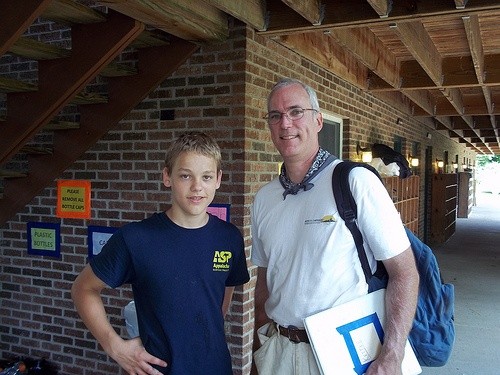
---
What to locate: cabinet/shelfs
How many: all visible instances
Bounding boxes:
[381,175,420,237]
[431,172,458,244]
[457,172,474,219]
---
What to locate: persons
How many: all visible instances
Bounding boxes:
[71,131,250,375]
[251,78,420,375]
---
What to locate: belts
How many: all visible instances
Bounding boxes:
[274,322,310,344]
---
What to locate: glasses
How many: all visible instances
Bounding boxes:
[263,106,319,125]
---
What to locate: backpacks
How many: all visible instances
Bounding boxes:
[332,161,455,367]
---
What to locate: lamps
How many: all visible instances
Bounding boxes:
[452,161,458,169]
[408,153,419,167]
[357,142,372,163]
[436,158,444,167]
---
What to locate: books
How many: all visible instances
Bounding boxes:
[303,289,422,375]
